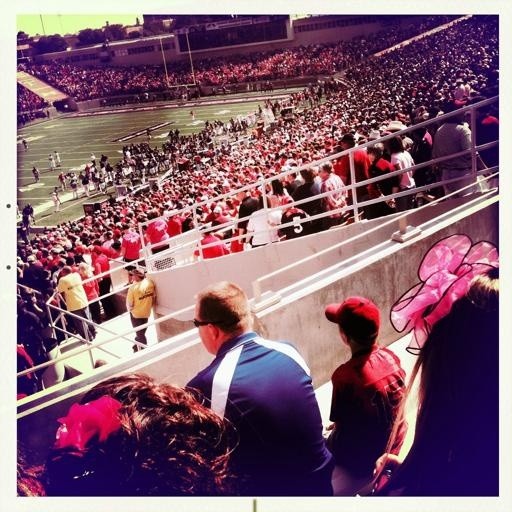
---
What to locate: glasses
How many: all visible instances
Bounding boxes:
[192,318,210,327]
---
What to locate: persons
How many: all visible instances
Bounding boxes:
[19,58,69,82]
[69,58,170,82]
[356,270,500,497]
[93,188,143,262]
[79,263,101,325]
[144,155,254,259]
[115,155,143,188]
[48,154,55,171]
[17,82,51,125]
[53,82,170,106]
[57,266,96,341]
[185,280,335,497]
[32,167,39,183]
[57,211,93,264]
[170,50,254,101]
[22,203,35,225]
[126,267,157,352]
[162,117,254,155]
[324,295,408,476]
[22,138,29,152]
[79,152,115,188]
[91,246,116,318]
[17,372,240,497]
[66,174,80,200]
[54,151,61,167]
[58,171,67,191]
[50,186,62,211]
[121,127,162,155]
[190,110,196,120]
[17,225,57,371]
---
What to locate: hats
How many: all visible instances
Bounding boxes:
[384,120,408,131]
[325,295,382,341]
[129,268,145,277]
